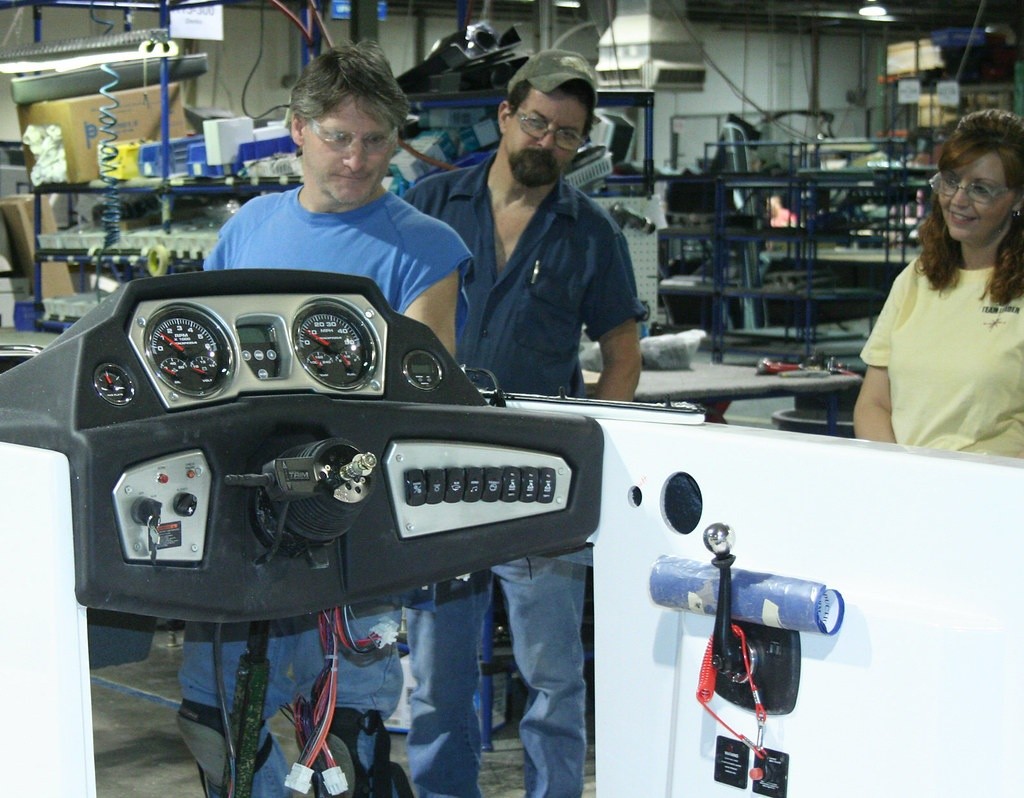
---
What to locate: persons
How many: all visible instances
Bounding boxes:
[854,105,1024,457]
[173,42,475,796]
[763,194,797,252]
[398,47,647,798]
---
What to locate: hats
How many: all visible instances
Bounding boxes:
[507,49,598,110]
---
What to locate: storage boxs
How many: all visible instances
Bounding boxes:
[1,194,76,298]
[16,84,189,188]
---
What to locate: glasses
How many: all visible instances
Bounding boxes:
[929,172,1023,203]
[509,105,585,151]
[304,115,397,154]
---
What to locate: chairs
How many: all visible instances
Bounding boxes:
[771,409,855,437]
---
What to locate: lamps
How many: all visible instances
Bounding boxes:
[858,1,888,19]
[0,28,181,74]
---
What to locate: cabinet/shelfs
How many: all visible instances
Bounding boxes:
[882,48,1024,251]
[714,171,907,363]
[28,177,304,335]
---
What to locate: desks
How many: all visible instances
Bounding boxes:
[577,364,863,438]
[1,328,64,355]
